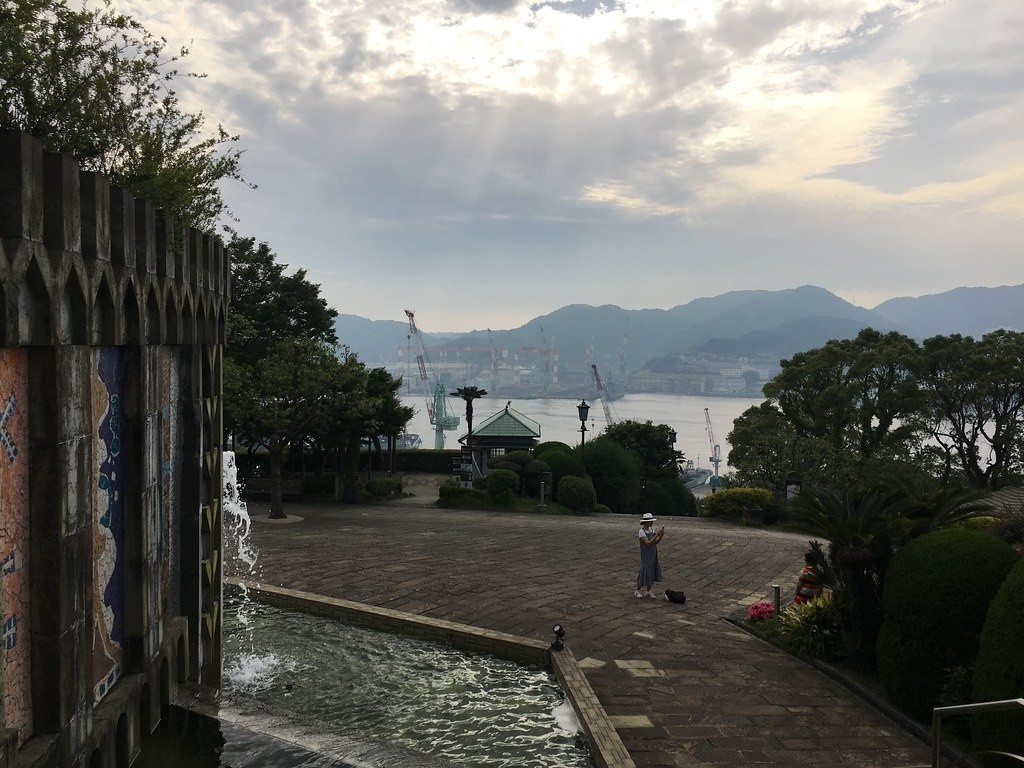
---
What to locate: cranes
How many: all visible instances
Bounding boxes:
[404,307,460,449]
[487,325,566,397]
[704,407,724,487]
[592,365,620,428]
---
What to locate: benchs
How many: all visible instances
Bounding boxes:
[242,477,305,504]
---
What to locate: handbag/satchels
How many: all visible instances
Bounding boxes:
[665,589,686,604]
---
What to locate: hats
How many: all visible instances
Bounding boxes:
[639,513,657,523]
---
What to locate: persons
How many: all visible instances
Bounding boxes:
[793,552,824,606]
[635,513,665,599]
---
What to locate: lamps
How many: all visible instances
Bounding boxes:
[551,624,565,651]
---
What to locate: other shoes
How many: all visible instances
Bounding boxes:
[635,591,643,597]
[645,591,655,598]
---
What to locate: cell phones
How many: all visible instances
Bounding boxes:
[662,525,665,530]
